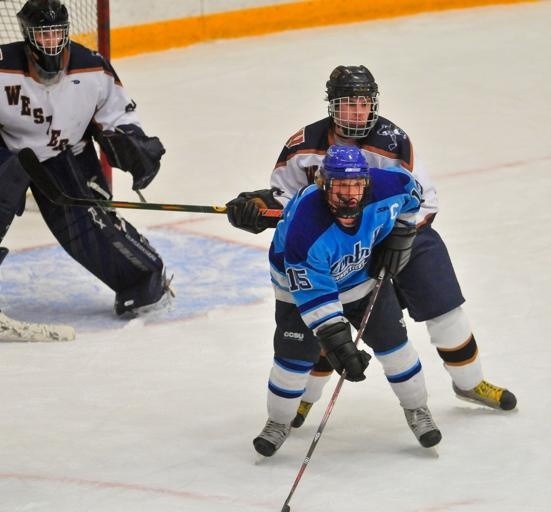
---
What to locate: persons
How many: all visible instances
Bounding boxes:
[251,143,443,457]
[0,0,177,322]
[226,62,519,430]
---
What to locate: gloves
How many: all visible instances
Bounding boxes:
[316,320,371,382]
[368,226,416,282]
[225,187,283,235]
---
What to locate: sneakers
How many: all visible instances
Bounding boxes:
[253,417,294,455]
[401,404,442,448]
[291,399,317,428]
[451,377,520,410]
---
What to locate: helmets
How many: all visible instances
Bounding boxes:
[325,63,380,138]
[317,142,375,221]
[17,0,69,64]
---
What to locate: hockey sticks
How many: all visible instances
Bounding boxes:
[282,265,383,512]
[18,147,282,219]
